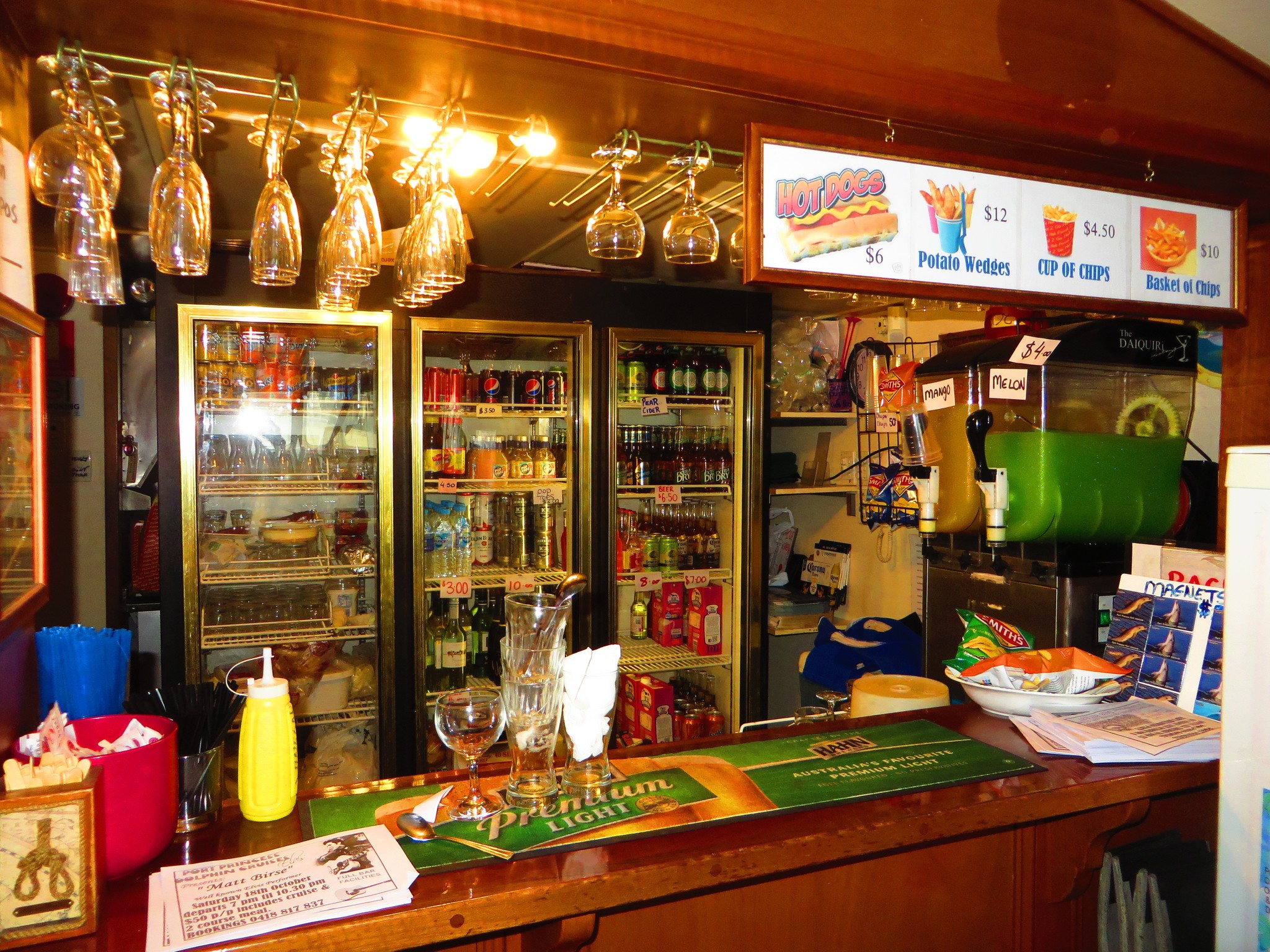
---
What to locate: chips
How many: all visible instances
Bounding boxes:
[878,360,923,431]
[943,608,1036,677]
[959,646,1135,694]
[865,463,919,533]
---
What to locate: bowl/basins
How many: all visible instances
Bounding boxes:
[11,713,180,886]
[1148,224,1188,264]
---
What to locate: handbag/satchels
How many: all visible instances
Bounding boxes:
[803,612,925,694]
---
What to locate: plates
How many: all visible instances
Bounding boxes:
[840,702,852,714]
[945,666,1121,717]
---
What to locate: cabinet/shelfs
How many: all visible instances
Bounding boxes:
[768,410,858,640]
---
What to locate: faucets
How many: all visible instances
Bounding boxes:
[903,409,943,542]
[964,407,1011,561]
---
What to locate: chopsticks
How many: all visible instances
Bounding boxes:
[121,679,247,819]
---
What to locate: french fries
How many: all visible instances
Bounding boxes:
[920,178,976,220]
[1042,204,1078,223]
[1145,218,1189,260]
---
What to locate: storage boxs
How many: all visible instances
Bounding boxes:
[1,765,104,950]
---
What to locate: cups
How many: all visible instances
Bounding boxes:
[178,741,224,833]
[881,354,943,466]
[202,431,375,636]
[793,707,835,724]
[847,679,857,709]
[497,593,621,805]
[1044,216,1074,257]
[927,198,974,254]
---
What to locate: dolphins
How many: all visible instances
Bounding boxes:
[1107,596,1222,721]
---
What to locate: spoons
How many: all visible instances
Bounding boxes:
[397,813,514,862]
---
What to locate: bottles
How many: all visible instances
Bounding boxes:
[424,414,734,758]
[228,647,299,822]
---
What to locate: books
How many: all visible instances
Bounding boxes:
[1005,689,1221,766]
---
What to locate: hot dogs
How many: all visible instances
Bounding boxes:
[780,194,898,262]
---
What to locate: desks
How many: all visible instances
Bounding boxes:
[0,707,1219,952]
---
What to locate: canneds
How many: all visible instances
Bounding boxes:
[456,492,557,570]
[616,360,648,404]
[423,367,568,413]
[674,698,725,741]
[196,321,372,413]
[631,532,678,577]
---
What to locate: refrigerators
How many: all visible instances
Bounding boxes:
[151,288,766,795]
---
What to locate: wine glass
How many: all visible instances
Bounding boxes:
[434,687,504,824]
[816,690,849,714]
[26,54,746,314]
